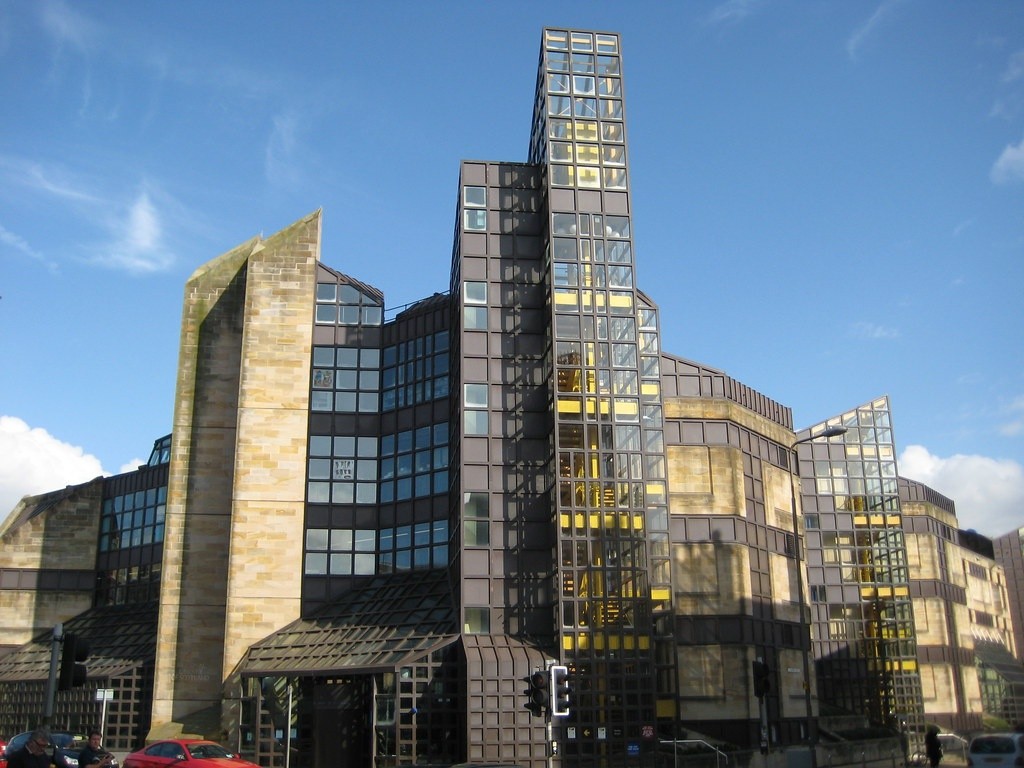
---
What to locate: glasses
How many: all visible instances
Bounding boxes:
[33,739,47,749]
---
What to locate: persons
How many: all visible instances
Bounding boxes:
[6,728,50,768]
[78,731,113,768]
[925,727,943,768]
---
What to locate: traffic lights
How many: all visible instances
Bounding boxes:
[550,665,572,716]
[531,671,549,708]
[523,675,542,717]
[52,631,91,694]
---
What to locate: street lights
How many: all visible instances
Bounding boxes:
[788,425,850,768]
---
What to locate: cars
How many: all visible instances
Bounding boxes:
[4,729,120,768]
[123,739,264,768]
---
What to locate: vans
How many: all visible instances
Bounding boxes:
[966,734,1023,768]
